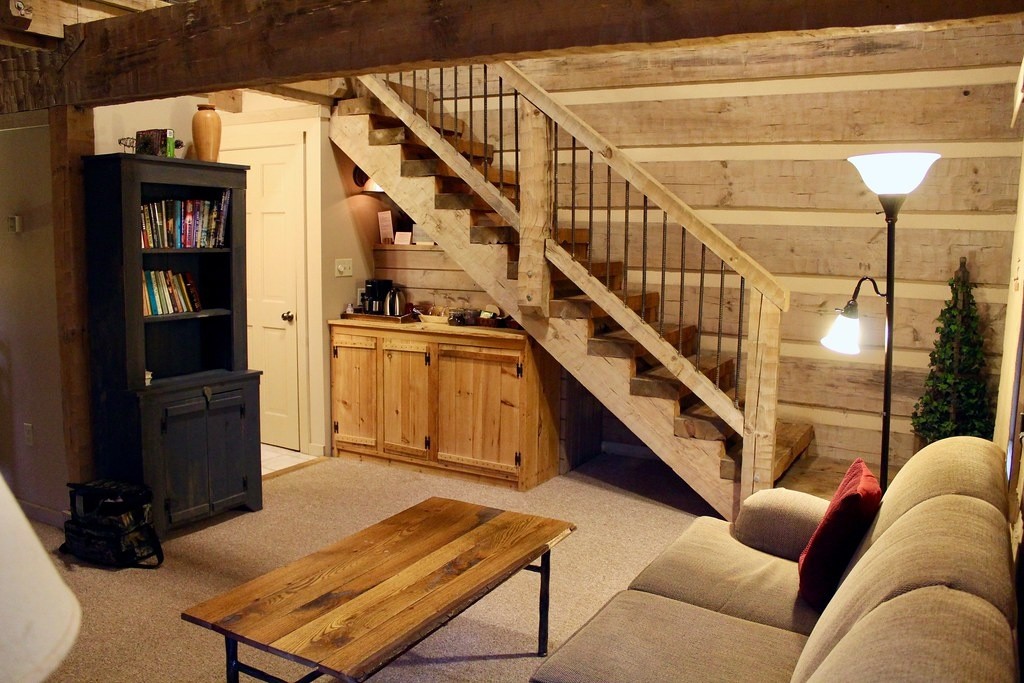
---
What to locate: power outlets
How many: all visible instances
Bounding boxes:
[357,288,365,306]
[24,423,32,446]
[335,259,352,277]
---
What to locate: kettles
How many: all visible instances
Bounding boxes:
[384,287,407,316]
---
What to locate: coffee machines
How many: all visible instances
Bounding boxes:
[360,279,392,314]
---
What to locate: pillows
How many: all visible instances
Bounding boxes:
[800,458,883,614]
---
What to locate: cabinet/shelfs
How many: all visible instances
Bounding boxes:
[81,152,265,543]
[328,317,560,492]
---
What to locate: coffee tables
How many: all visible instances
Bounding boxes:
[181,496,577,683]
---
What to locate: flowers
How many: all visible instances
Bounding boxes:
[175,140,184,149]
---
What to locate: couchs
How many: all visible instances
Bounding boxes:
[529,436,1020,683]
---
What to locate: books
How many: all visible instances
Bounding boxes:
[140,190,230,316]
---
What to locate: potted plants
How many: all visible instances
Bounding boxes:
[910,257,999,456]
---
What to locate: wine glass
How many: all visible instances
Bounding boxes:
[427,291,440,316]
[440,293,452,317]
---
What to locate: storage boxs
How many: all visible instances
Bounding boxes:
[136,130,174,158]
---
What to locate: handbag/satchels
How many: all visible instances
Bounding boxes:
[58,475,165,570]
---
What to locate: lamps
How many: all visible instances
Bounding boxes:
[819,153,944,495]
[353,165,384,192]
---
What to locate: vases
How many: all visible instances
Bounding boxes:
[192,104,222,162]
[174,148,183,157]
